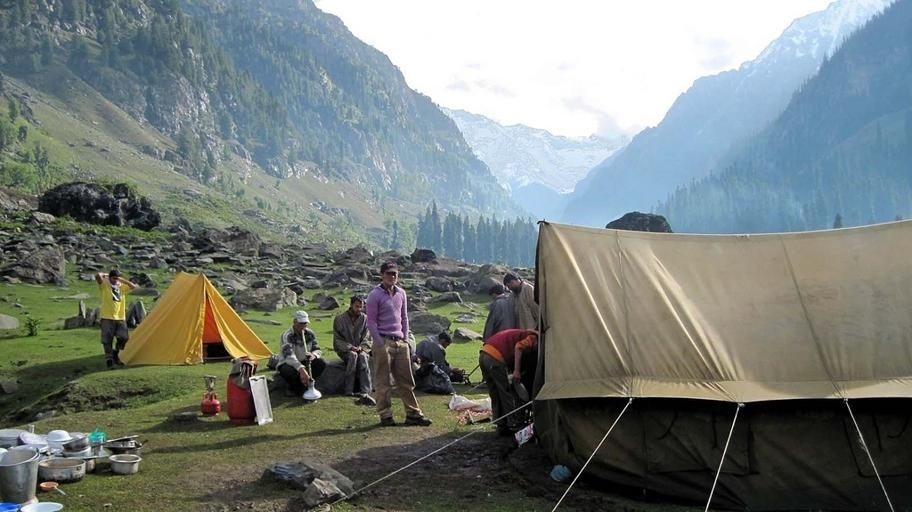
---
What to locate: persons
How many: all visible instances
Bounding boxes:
[333,294,374,398]
[94,269,137,367]
[503,271,540,329]
[416,333,464,382]
[276,310,326,403]
[479,284,516,381]
[479,328,539,437]
[365,261,432,425]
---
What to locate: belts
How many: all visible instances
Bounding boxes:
[379,332,403,342]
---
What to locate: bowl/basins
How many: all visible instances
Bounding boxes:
[173,412,197,421]
[0,429,146,482]
[0,499,65,512]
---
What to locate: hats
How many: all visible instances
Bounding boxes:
[380,262,399,274]
[293,310,311,325]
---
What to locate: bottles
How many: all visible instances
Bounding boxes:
[302,361,323,402]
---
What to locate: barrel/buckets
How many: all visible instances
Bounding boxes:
[88,431,105,449]
[0,448,41,505]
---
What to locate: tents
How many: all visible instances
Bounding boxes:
[117,271,273,368]
[534,218,911,512]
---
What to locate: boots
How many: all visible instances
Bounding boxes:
[105,350,124,371]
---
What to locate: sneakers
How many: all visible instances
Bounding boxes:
[381,416,395,426]
[360,394,376,405]
[404,415,432,427]
